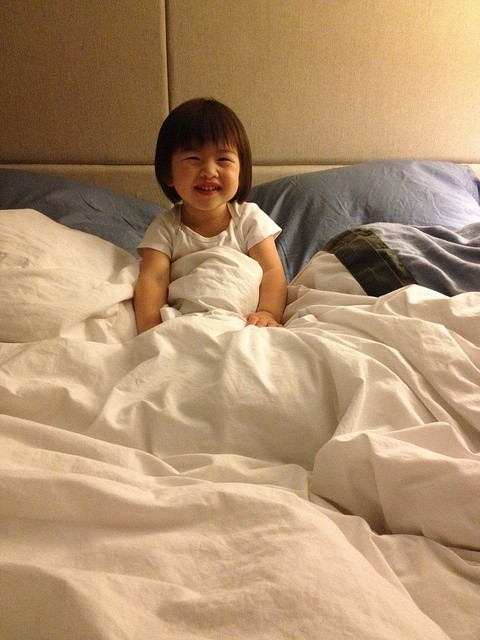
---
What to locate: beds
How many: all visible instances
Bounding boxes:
[0,164,480,640]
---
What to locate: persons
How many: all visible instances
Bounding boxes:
[134,98,288,334]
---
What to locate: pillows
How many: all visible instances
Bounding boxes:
[0,166,167,260]
[243,161,480,285]
